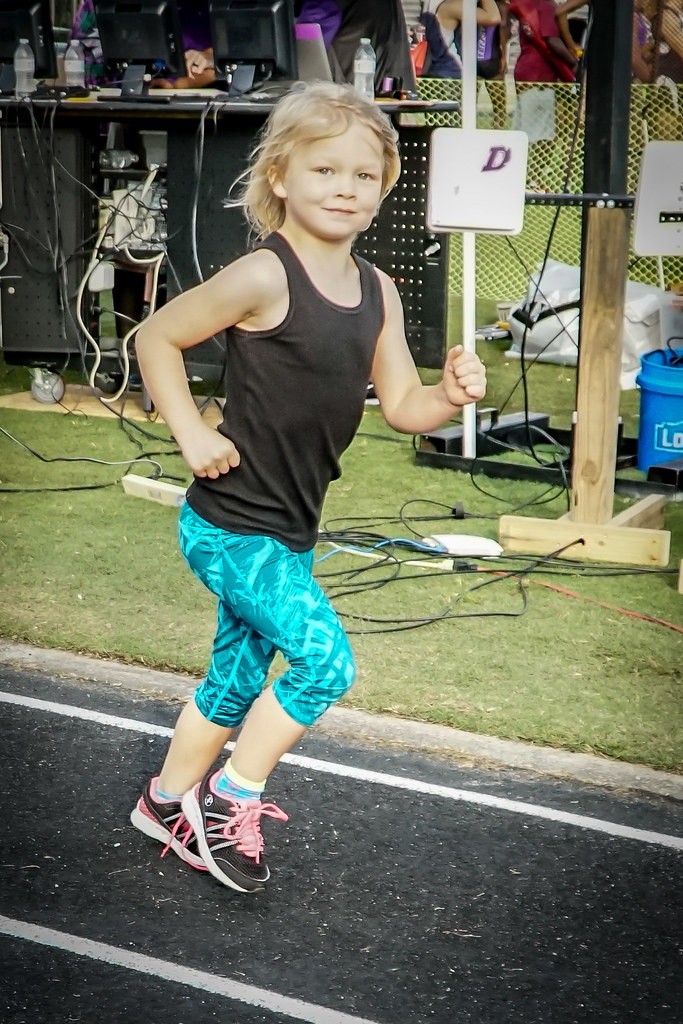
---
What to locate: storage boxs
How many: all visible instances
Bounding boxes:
[657,290,683,350]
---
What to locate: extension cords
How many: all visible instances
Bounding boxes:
[121,474,195,507]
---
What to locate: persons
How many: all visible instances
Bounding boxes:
[130,76,486,892]
[147,1,682,198]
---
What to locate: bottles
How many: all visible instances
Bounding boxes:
[352,37,376,101]
[98,149,139,169]
[65,40,85,89]
[14,39,36,100]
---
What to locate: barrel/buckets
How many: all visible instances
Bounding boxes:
[634,347,683,475]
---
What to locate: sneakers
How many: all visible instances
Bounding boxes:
[181,769,289,893]
[131,777,209,871]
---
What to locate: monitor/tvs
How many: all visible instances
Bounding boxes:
[0,0,59,80]
[208,0,300,81]
[93,0,189,78]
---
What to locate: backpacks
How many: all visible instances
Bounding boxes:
[410,0,449,77]
[477,22,501,79]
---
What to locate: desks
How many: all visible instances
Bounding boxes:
[0,98,463,414]
